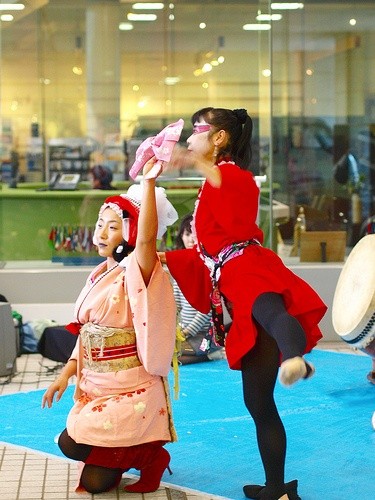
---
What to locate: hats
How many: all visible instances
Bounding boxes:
[91,184,179,249]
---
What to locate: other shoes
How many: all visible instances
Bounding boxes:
[242,479,301,500]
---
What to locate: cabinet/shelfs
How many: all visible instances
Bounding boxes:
[49,138,90,180]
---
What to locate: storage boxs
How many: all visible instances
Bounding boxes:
[300,230,347,262]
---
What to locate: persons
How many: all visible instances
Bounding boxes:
[41,155,179,494]
[86,164,117,190]
[155,107,328,500]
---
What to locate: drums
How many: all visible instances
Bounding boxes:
[331,233,375,357]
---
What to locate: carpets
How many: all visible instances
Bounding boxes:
[0,349,375,500]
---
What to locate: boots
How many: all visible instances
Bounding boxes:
[84,441,173,493]
